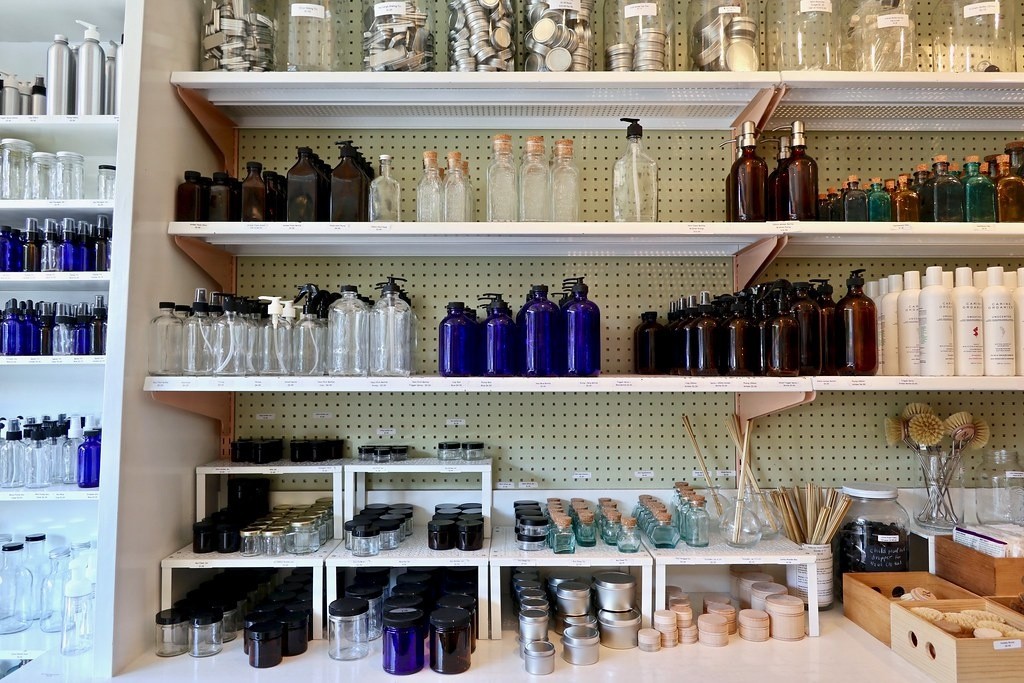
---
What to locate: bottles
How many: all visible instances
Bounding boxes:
[838,480,909,601]
[326,286,377,377]
[515,286,559,376]
[553,139,580,222]
[0,529,97,654]
[486,134,516,222]
[544,480,781,554]
[44,19,124,116]
[146,302,221,375]
[521,133,552,222]
[176,147,330,222]
[629,292,721,375]
[819,141,1024,223]
[417,149,475,223]
[368,155,401,222]
[435,300,478,377]
[976,447,1024,527]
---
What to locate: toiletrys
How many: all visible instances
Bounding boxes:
[631,264,1024,377]
[724,119,1024,224]
[173,140,376,223]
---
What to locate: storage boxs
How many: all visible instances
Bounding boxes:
[842,572,982,648]
[891,600,1024,683]
[934,534,1024,596]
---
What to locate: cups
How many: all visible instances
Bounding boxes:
[1,138,34,200]
[152,569,313,667]
[56,153,84,200]
[853,0,915,70]
[767,0,842,72]
[98,164,115,200]
[201,0,762,72]
[329,566,478,674]
[192,478,332,556]
[347,502,485,555]
[29,152,55,199]
[786,543,837,610]
[513,499,549,550]
[931,0,1014,73]
[229,439,485,463]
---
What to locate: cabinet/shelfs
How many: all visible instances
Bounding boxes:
[0,71,1024,683]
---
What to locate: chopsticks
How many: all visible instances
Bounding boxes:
[772,484,852,544]
